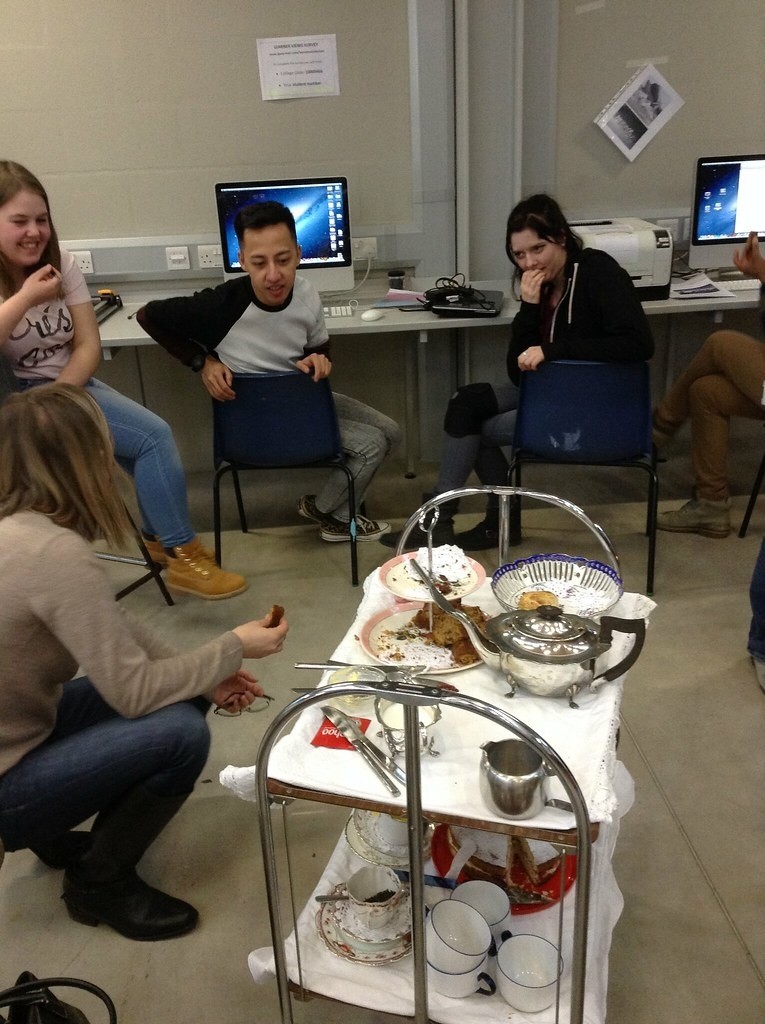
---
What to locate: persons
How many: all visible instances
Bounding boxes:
[0,161,248,599]
[137,201,402,544]
[654,231,765,539]
[0,383,287,940]
[378,192,655,549]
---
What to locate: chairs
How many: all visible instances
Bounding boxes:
[0,350,176,610]
[504,357,658,596]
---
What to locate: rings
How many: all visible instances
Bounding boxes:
[522,352,526,356]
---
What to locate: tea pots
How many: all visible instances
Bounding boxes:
[448,606,646,709]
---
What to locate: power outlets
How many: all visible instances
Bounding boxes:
[684,218,690,240]
[198,244,222,269]
[73,251,94,274]
[352,237,378,261]
[165,246,190,270]
[657,219,679,241]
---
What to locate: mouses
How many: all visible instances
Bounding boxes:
[361,310,384,322]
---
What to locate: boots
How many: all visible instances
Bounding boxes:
[378,494,456,549]
[28,831,89,870]
[62,781,199,942]
[455,493,522,550]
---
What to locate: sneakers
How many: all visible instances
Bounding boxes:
[652,427,678,462]
[140,527,248,600]
[657,486,733,538]
[298,495,391,542]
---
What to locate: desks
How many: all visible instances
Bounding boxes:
[81,268,761,481]
[209,371,365,587]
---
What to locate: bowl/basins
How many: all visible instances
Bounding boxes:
[491,552,624,617]
[326,664,386,714]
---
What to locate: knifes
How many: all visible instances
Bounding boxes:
[411,558,471,624]
[289,660,460,796]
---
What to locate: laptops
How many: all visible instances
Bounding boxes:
[432,290,504,316]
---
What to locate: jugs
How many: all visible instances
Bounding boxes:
[480,738,573,819]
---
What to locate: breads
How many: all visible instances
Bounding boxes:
[405,543,472,584]
[412,597,489,665]
[269,604,285,627]
[518,591,559,611]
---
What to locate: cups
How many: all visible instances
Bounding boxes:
[427,953,496,999]
[347,864,402,930]
[496,931,564,1013]
[424,899,492,974]
[448,880,510,940]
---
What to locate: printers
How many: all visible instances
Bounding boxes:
[571,218,673,300]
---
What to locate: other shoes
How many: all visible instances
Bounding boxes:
[752,654,765,694]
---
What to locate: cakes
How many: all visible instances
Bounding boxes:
[448,825,564,885]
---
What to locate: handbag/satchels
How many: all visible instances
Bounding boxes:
[0,971,117,1024]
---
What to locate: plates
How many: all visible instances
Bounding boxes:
[431,824,577,915]
[315,882,426,968]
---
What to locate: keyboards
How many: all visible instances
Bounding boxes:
[323,306,352,317]
[715,279,763,292]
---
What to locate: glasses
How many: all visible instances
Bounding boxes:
[213,692,275,717]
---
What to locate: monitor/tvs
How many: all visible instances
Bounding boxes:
[688,154,765,279]
[215,176,355,292]
